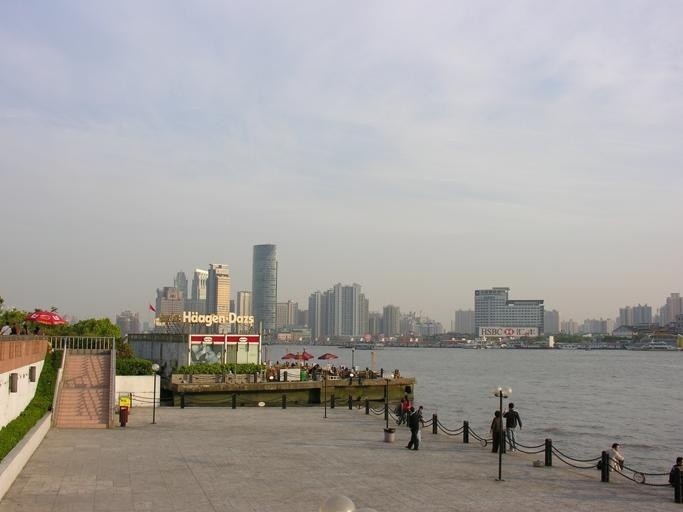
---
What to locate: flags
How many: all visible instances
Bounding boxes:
[148,304,156,312]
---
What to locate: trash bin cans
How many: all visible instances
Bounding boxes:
[313,369,321,381]
[384,428,396,442]
[300,371,306,381]
[119,406,128,426]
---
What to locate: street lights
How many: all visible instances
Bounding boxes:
[490,386,512,481]
[151,363,160,424]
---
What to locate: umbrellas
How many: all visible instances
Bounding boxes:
[26,311,64,335]
[281,351,338,370]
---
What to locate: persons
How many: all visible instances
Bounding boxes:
[489,402,523,452]
[669,457,683,487]
[396,395,424,450]
[266,360,374,382]
[601,443,624,471]
[191,344,221,365]
[0,321,41,335]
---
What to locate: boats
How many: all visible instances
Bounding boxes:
[641,341,674,351]
[343,341,383,349]
